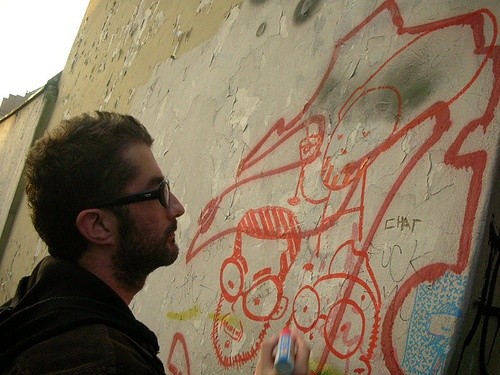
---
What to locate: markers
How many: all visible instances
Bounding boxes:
[274,325,296,374]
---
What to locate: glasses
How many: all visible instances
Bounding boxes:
[81,180,171,214]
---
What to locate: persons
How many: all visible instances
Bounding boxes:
[1,110,311,375]
